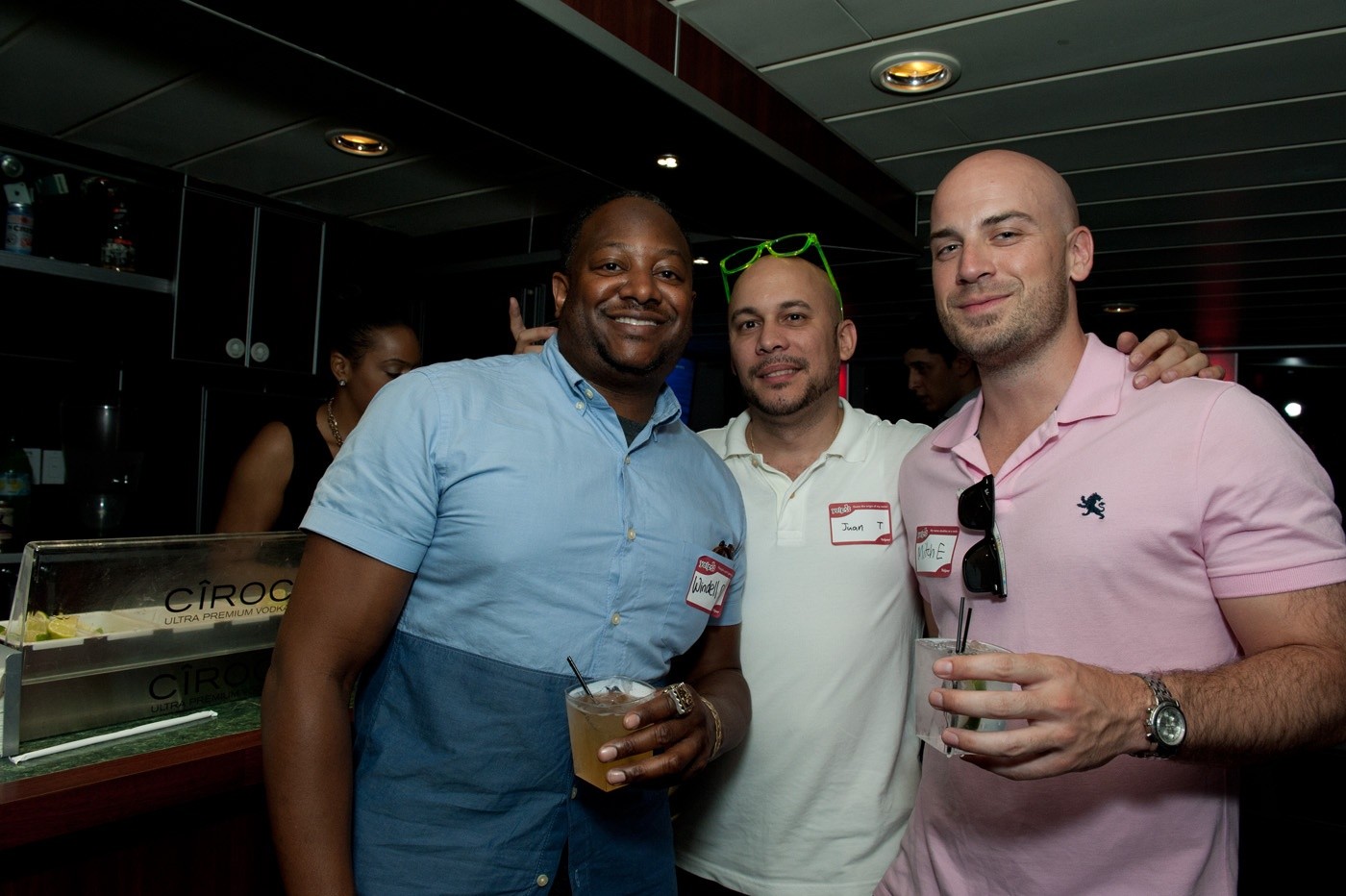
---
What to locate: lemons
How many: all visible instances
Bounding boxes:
[257,589,289,607]
[958,679,987,730]
[19,611,104,642]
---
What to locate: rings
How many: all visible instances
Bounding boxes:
[662,682,695,714]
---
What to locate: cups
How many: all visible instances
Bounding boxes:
[564,675,657,792]
[912,637,1015,758]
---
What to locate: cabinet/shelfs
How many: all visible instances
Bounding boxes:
[170,188,422,378]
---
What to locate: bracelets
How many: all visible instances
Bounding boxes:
[701,697,722,763]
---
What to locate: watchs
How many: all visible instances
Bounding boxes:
[1129,673,1187,759]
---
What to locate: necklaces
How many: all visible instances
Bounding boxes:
[326,397,343,447]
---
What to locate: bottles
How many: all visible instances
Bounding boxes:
[5,202,34,257]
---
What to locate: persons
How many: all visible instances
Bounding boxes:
[261,200,751,896]
[884,310,982,425]
[508,232,1225,896]
[207,316,423,596]
[898,151,1346,896]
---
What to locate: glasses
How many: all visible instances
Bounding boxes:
[719,231,845,317]
[957,473,1008,598]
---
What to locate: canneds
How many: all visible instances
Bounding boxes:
[5,202,34,255]
